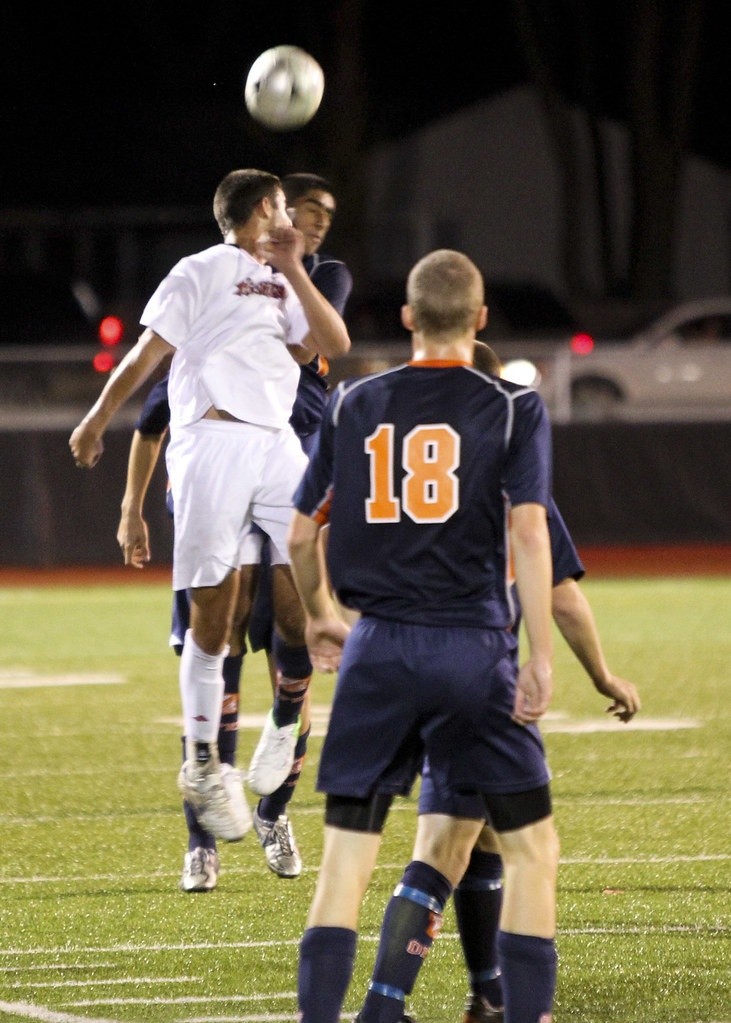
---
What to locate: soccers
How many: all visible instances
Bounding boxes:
[244,46,326,129]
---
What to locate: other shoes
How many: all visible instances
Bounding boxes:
[465,995,504,1023]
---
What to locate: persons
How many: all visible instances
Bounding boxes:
[69,170,354,890]
[288,248,643,1023]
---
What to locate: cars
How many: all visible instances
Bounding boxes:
[538,300,731,427]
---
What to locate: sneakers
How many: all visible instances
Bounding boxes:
[182,846,217,891]
[177,759,251,842]
[248,708,302,796]
[252,804,302,878]
